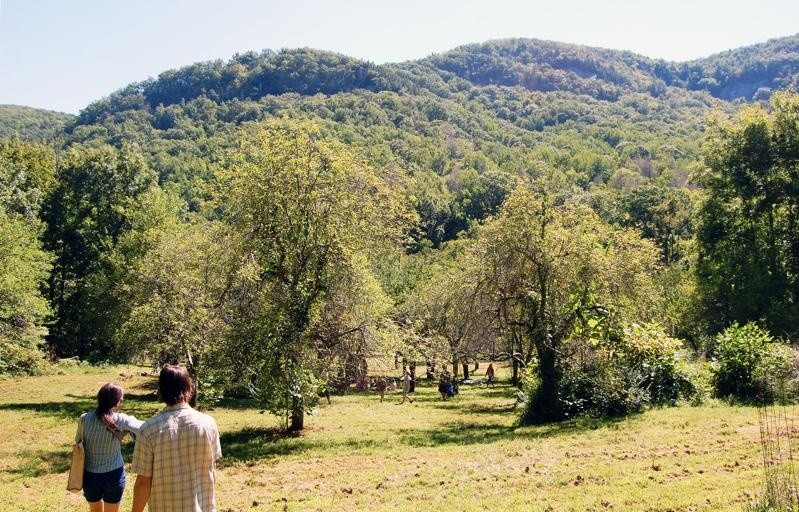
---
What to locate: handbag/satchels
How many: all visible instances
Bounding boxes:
[66,440,86,494]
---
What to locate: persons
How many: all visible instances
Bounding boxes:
[75,382,145,512]
[324,351,419,405]
[426,351,470,401]
[484,363,495,388]
[132,363,222,512]
